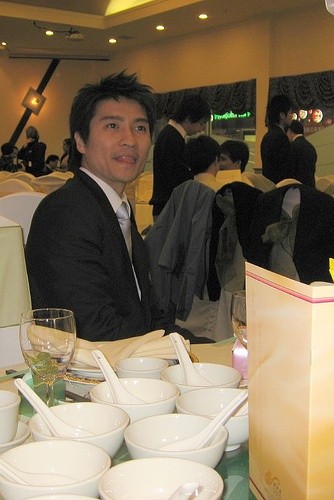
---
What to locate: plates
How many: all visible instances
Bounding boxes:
[0,421,31,453]
[62,341,116,380]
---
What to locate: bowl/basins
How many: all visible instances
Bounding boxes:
[0,440,112,500]
[175,387,248,452]
[123,413,228,469]
[29,402,130,460]
[90,378,180,425]
[160,362,242,395]
[97,457,224,500]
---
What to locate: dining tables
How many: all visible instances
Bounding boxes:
[0,342,249,500]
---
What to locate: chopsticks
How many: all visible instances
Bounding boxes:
[6,369,91,401]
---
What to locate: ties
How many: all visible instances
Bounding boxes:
[116,202,133,267]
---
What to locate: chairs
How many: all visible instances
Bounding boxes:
[0,170,334,370]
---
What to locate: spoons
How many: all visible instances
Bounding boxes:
[14,378,96,437]
[158,388,248,452]
[0,457,79,486]
[90,350,148,405]
[169,333,213,386]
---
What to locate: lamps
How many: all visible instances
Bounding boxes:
[64,30,84,41]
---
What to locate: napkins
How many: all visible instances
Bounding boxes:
[26,324,191,372]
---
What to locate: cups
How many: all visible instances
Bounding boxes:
[231,290,247,349]
[0,390,21,444]
[19,308,76,407]
[115,357,169,380]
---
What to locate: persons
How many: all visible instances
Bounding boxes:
[17,126,46,177]
[11,147,18,165]
[41,155,60,176]
[285,119,318,187]
[0,143,17,173]
[149,96,210,222]
[218,139,254,187]
[60,138,72,172]
[25,68,214,343]
[186,135,224,192]
[260,94,296,183]
